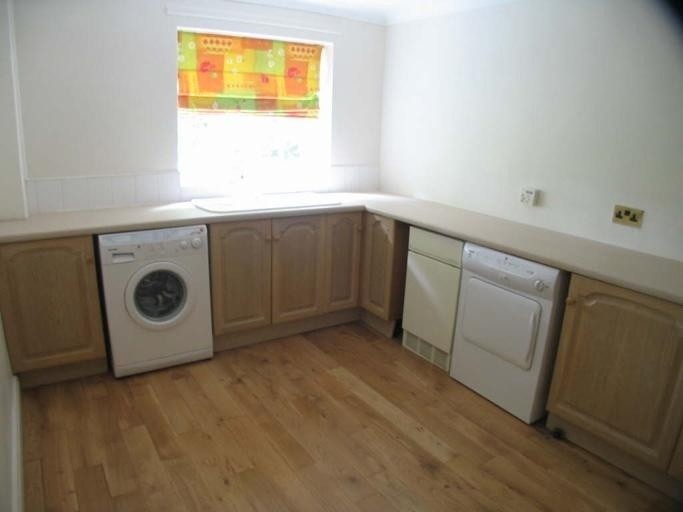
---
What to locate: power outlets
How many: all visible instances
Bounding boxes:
[612,205,644,228]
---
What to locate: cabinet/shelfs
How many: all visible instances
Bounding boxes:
[546,274,683,472]
[325,214,393,317]
[0,236,107,375]
[210,214,326,337]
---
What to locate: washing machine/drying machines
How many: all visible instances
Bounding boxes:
[98,224,214,380]
